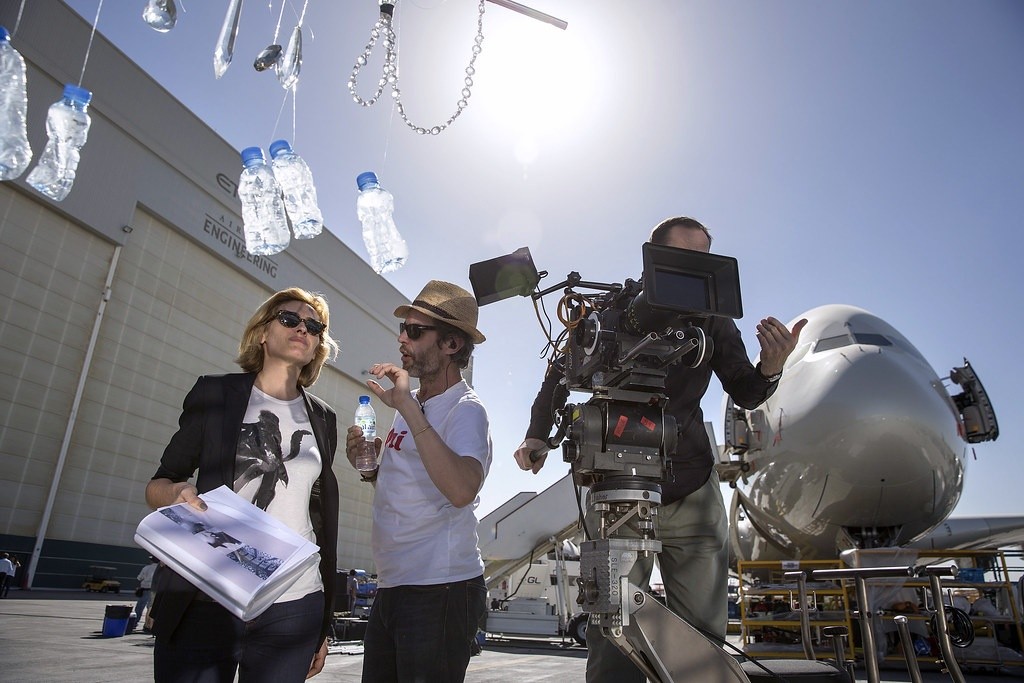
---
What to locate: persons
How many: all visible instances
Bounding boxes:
[346,570,359,623]
[0,553,22,599]
[132,555,161,630]
[359,279,493,683]
[513,215,809,683]
[144,288,340,683]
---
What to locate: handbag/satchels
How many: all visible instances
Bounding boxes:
[135,588,144,598]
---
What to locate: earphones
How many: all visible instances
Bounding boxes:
[450,340,457,349]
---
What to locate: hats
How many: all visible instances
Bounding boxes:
[393,280,486,344]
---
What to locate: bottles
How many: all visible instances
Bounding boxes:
[26,82,92,202]
[238,146,291,256]
[356,171,410,275]
[354,396,378,471]
[0,26,33,181]
[269,139,323,240]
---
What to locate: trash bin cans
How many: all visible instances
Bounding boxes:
[103,603,134,637]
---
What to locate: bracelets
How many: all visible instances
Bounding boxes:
[361,464,378,482]
[414,424,432,439]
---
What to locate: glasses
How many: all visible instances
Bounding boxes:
[400,322,461,341]
[265,309,326,338]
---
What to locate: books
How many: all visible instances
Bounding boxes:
[133,485,320,623]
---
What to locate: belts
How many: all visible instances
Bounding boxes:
[144,588,151,591]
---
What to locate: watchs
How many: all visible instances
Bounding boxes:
[757,361,783,383]
